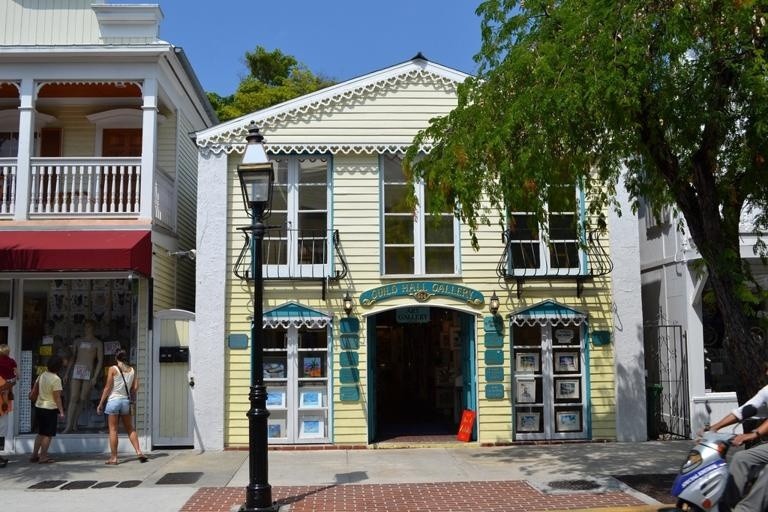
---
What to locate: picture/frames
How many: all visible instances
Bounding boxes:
[433,326,463,410]
[262,356,325,439]
[514,352,580,433]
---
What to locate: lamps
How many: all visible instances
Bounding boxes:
[488,290,499,315]
[343,290,352,317]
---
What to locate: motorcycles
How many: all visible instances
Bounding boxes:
[656,400,767,512]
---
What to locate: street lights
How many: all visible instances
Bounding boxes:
[236,119,280,511]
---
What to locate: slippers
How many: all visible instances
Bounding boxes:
[30,457,55,463]
[106,460,117,464]
[138,454,148,462]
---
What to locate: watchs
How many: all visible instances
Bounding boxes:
[751,429,760,437]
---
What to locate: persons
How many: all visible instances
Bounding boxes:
[60,320,103,433]
[36,319,66,377]
[29,356,65,464]
[102,320,130,368]
[97,349,149,465]
[697,356,768,512]
[0,377,15,468]
[0,344,18,411]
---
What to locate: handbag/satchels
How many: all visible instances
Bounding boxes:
[30,381,39,402]
[131,402,135,415]
